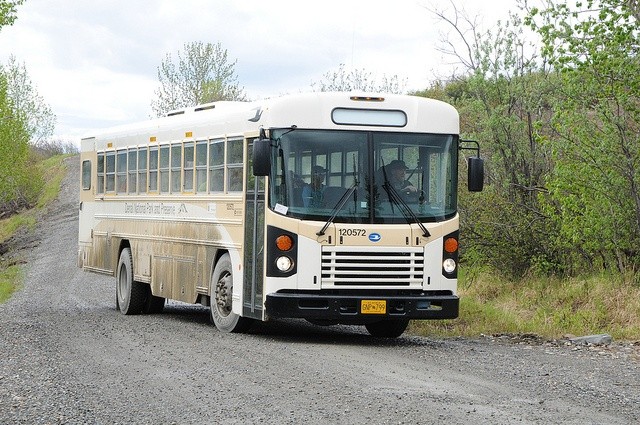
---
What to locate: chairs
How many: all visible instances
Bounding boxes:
[322,186,347,209]
[297,183,308,206]
[281,170,295,199]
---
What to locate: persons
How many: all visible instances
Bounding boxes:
[377,160,418,203]
[301,166,328,207]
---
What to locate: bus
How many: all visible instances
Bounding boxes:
[77,91,484,338]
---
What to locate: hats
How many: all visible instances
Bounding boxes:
[312,166,328,172]
[391,160,409,169]
